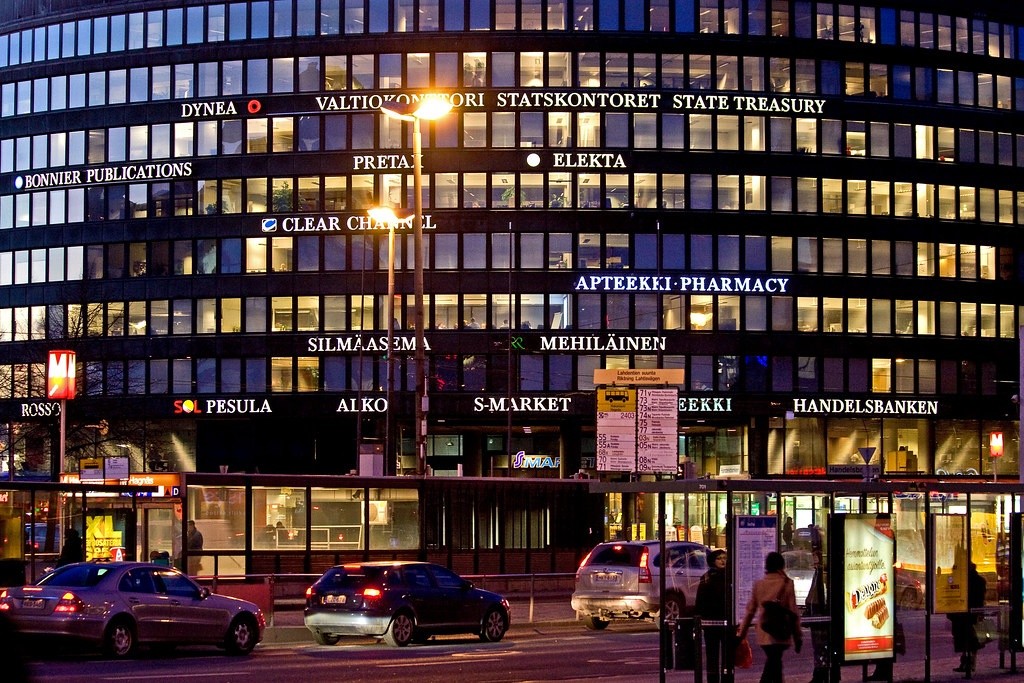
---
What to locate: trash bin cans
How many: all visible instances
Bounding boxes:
[660,616,697,671]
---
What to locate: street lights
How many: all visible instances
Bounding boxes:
[989,430,1004,482]
[410,89,452,475]
[366,206,400,475]
[46,349,77,483]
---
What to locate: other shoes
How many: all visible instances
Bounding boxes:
[867,670,891,680]
[952,654,974,673]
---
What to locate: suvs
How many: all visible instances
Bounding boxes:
[571,538,718,630]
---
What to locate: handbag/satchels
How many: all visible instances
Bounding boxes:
[761,579,800,642]
[894,618,908,655]
[802,604,811,627]
[730,627,753,669]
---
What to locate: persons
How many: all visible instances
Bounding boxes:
[437,317,512,330]
[695,516,985,683]
[181,520,204,578]
[55,527,84,570]
[260,521,290,541]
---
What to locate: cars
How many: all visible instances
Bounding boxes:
[303,560,510,648]
[0,561,265,657]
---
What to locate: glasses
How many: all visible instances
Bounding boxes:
[716,557,727,559]
